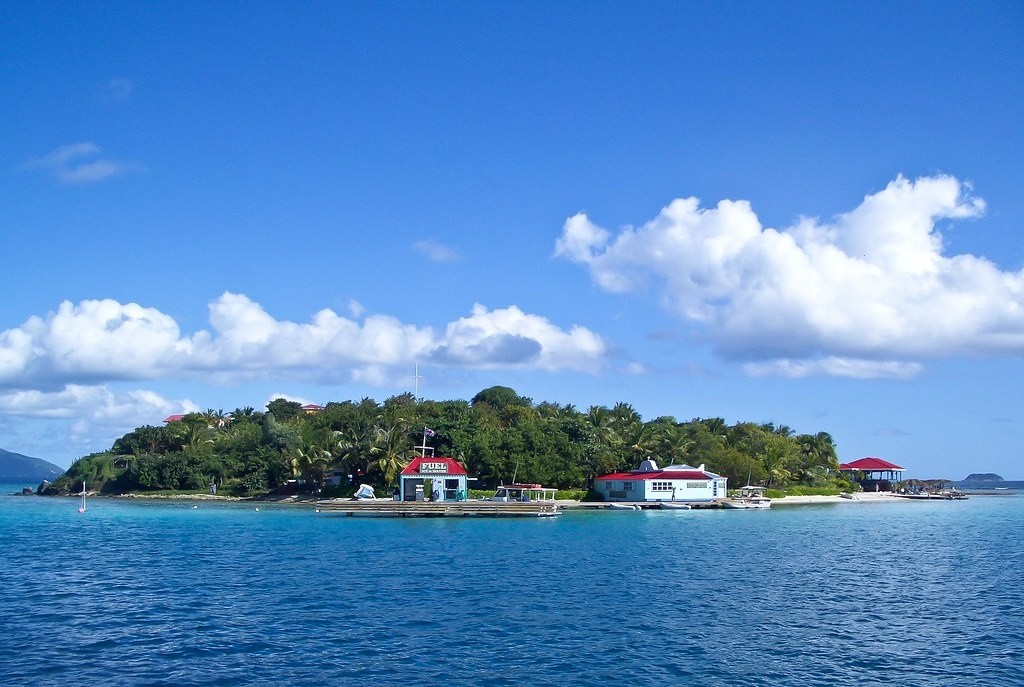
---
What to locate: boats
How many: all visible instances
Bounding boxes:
[477,461,561,515]
[721,469,771,508]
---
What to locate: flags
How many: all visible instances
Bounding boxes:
[424,428,436,437]
